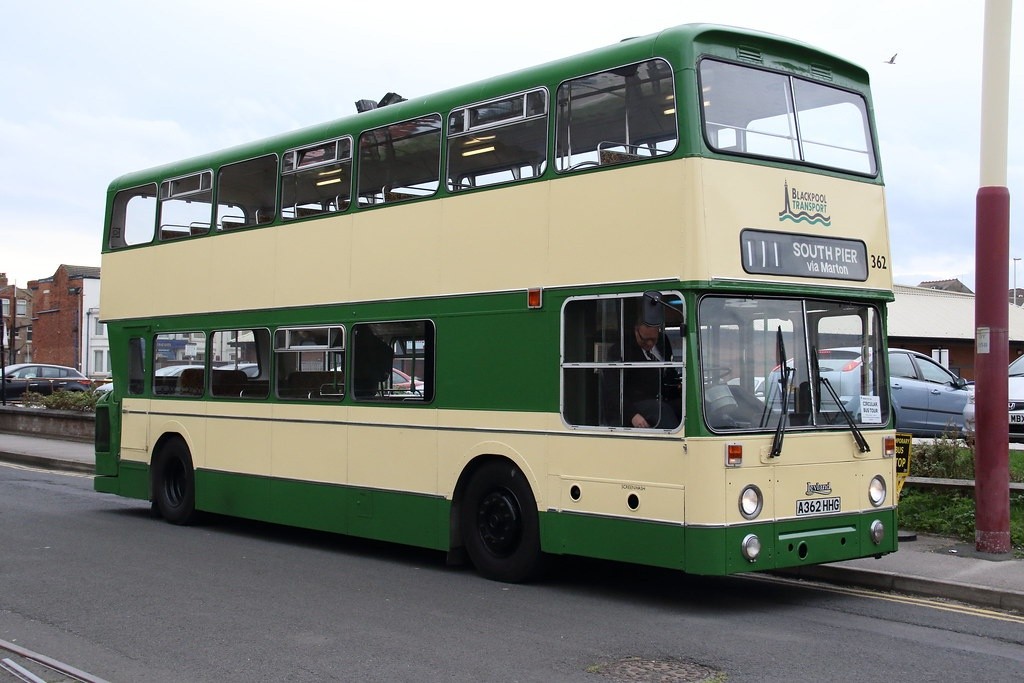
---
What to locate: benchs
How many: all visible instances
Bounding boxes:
[130,140,670,401]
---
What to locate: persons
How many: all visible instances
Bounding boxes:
[601,313,680,428]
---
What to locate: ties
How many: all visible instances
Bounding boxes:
[646,349,660,362]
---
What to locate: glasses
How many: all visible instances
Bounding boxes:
[637,329,658,343]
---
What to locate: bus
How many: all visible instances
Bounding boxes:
[94,22,899,583]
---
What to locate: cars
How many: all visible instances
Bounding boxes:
[215,363,259,378]
[727,377,766,403]
[330,366,424,389]
[765,347,969,438]
[962,354,1024,447]
[92,365,216,398]
[0,364,98,401]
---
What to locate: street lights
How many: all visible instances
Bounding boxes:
[1013,257,1022,305]
